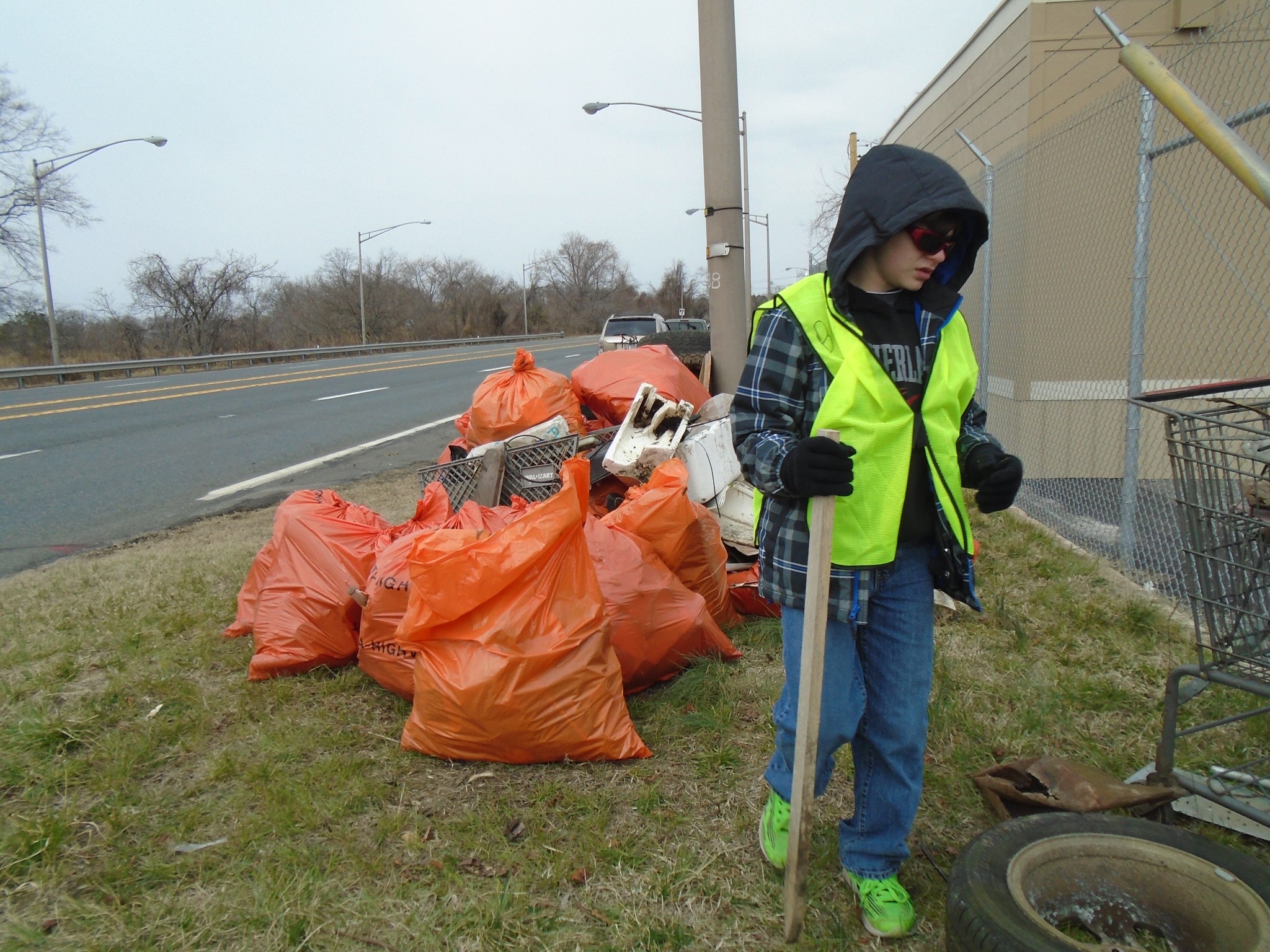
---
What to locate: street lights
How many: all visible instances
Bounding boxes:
[358,221,432,348]
[32,136,168,383]
[583,103,752,342]
[687,207,773,302]
[523,255,569,342]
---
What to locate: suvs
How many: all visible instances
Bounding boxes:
[597,314,708,361]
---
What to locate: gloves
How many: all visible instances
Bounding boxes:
[960,443,1023,514]
[787,437,856,497]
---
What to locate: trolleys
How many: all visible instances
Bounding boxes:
[1126,376,1270,830]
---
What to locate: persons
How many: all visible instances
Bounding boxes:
[730,144,1022,938]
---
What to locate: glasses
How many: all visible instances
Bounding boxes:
[901,226,960,258]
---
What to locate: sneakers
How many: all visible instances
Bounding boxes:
[759,789,791,868]
[844,868,917,938]
